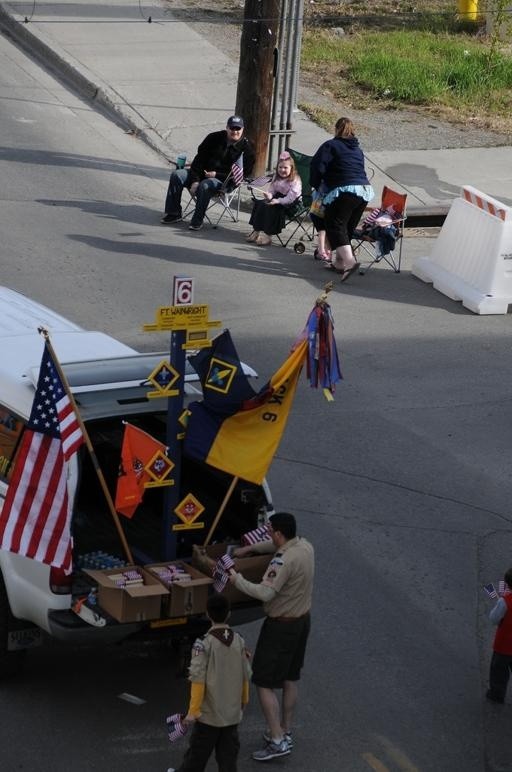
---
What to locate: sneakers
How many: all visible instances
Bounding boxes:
[188,218,203,230]
[160,214,182,224]
[316,249,331,260]
[252,739,292,761]
[263,728,294,749]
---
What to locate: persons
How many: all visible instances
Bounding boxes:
[488,568,512,703]
[161,114,254,231]
[310,185,331,261]
[229,514,314,762]
[247,151,302,246]
[169,595,253,772]
[310,116,375,282]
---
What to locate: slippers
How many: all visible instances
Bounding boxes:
[330,263,344,274]
[341,262,360,281]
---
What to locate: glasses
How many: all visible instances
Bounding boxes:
[229,126,241,131]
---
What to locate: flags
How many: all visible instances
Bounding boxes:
[232,155,244,185]
[212,522,273,593]
[364,206,403,226]
[185,305,323,485]
[483,584,497,599]
[117,425,162,519]
[0,344,83,576]
[498,581,509,593]
[249,176,272,187]
[165,713,185,743]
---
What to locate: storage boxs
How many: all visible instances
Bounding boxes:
[191,543,278,626]
[79,563,170,624]
[141,558,214,618]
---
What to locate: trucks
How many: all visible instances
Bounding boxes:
[0,281,286,686]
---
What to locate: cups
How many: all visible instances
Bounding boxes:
[175,156,187,171]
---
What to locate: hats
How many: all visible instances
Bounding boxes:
[227,115,244,128]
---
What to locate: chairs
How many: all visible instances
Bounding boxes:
[247,146,319,247]
[351,184,409,274]
[180,137,256,231]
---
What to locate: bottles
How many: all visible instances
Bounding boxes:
[77,550,130,574]
[88,585,98,604]
